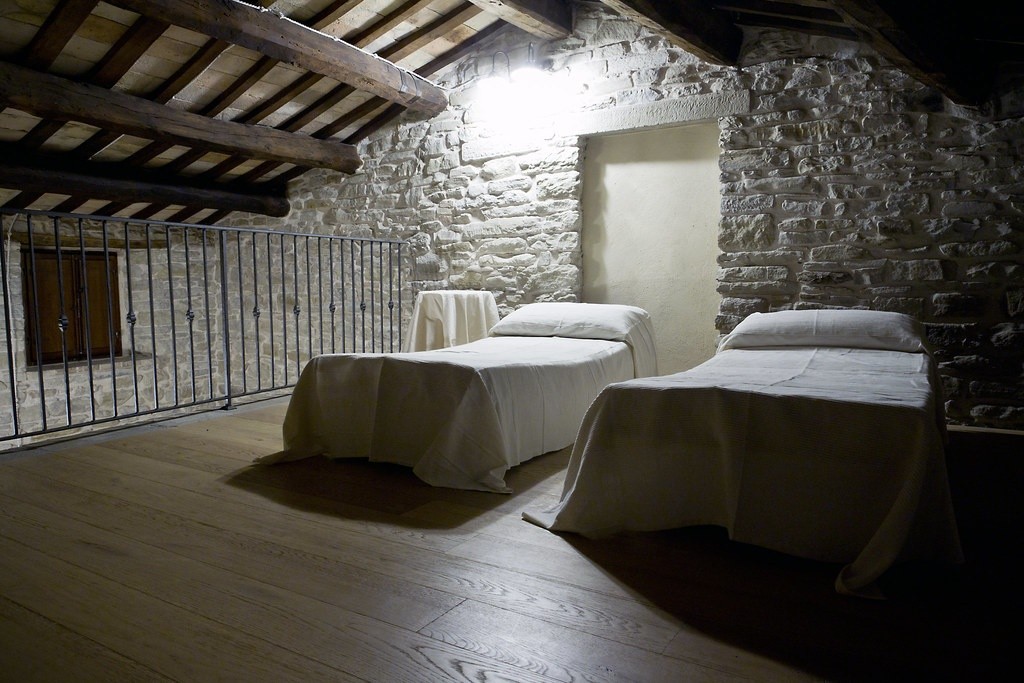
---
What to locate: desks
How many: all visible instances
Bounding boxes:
[399,291,500,354]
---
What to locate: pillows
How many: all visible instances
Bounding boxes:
[725,310,927,354]
[490,303,651,341]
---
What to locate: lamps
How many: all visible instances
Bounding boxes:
[473,42,581,121]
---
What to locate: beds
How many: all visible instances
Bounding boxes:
[282,301,662,495]
[522,309,963,602]
